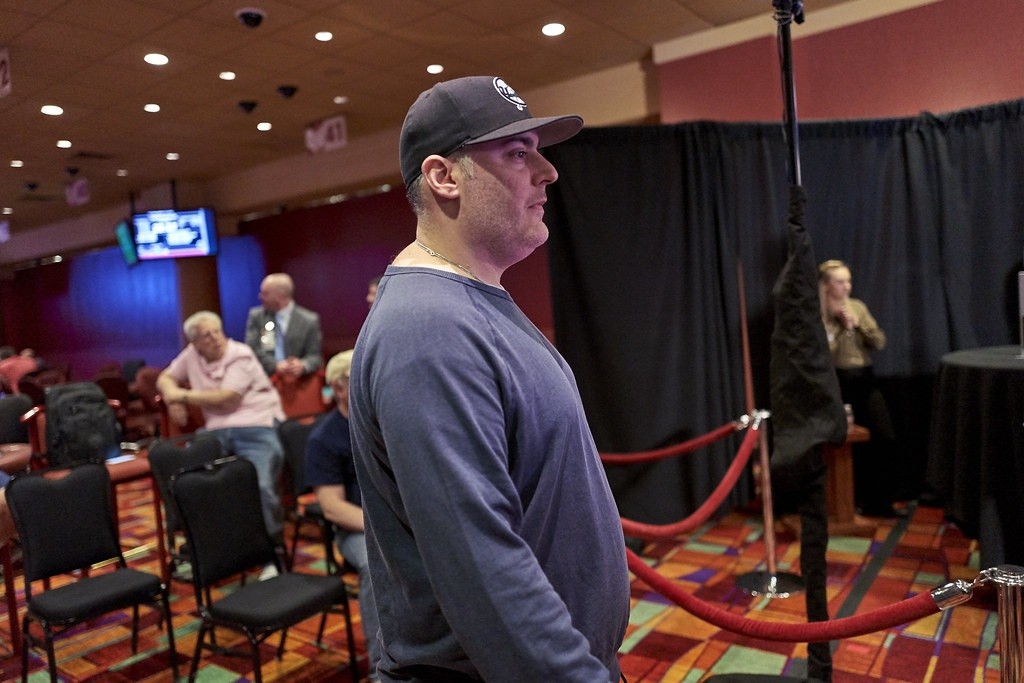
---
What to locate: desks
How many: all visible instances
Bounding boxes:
[934,344,1023,573]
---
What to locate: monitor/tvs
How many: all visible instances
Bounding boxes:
[130,205,220,262]
[114,218,138,272]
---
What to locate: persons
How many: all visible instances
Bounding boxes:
[819,260,909,518]
[349,75,631,683]
[156,310,287,582]
[245,273,322,377]
[365,270,385,310]
[304,350,385,683]
[123,361,148,401]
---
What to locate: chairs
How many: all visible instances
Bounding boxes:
[0,358,361,683]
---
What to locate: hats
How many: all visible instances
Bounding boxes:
[399,76,584,191]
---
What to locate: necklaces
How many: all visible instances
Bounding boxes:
[417,241,483,284]
